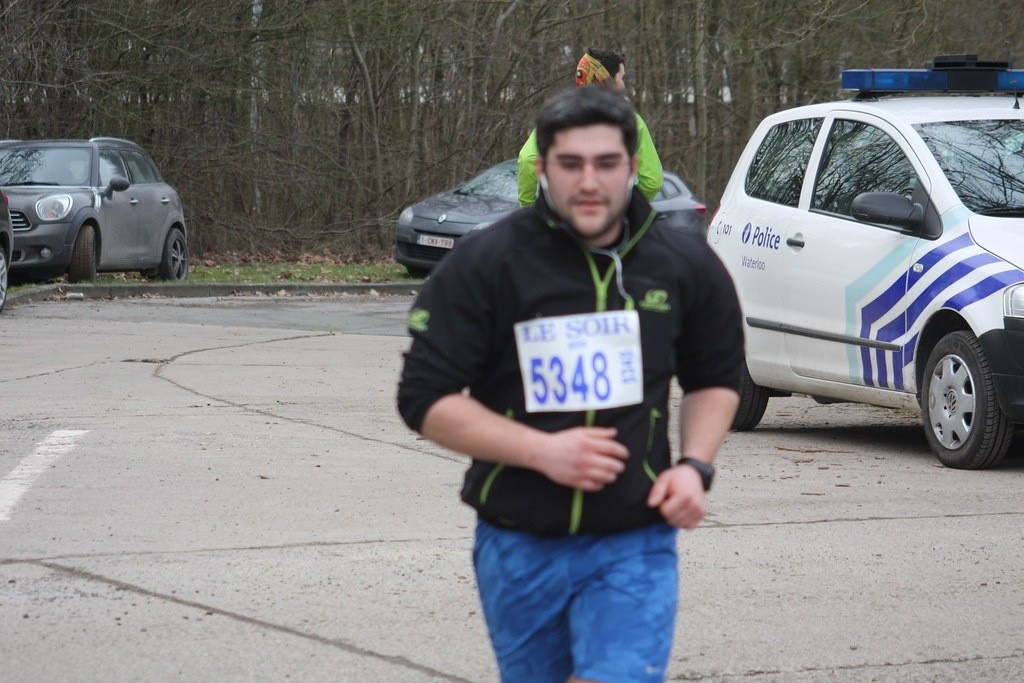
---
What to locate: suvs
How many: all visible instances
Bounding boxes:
[0,136,190,284]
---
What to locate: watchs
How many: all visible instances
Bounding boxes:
[678,458,714,491]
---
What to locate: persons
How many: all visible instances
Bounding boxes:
[394,85,745,683]
[518,47,664,208]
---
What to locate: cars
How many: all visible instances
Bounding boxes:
[389,157,705,279]
[704,54,1024,474]
[0,190,15,314]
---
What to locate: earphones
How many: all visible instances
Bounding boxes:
[627,175,635,193]
[539,172,548,191]
